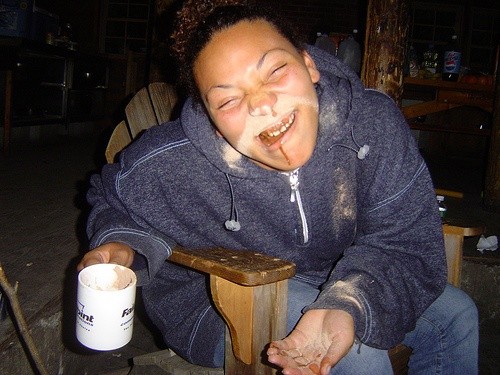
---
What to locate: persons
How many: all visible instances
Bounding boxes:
[77,0,479,375]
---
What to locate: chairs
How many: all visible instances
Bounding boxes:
[105,82,488,375]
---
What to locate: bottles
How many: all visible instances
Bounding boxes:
[314,29,363,74]
[436,195,449,225]
[407,35,467,82]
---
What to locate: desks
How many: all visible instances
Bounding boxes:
[402,76,500,201]
[0,37,128,150]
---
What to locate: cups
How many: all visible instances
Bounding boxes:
[75,262,136,352]
